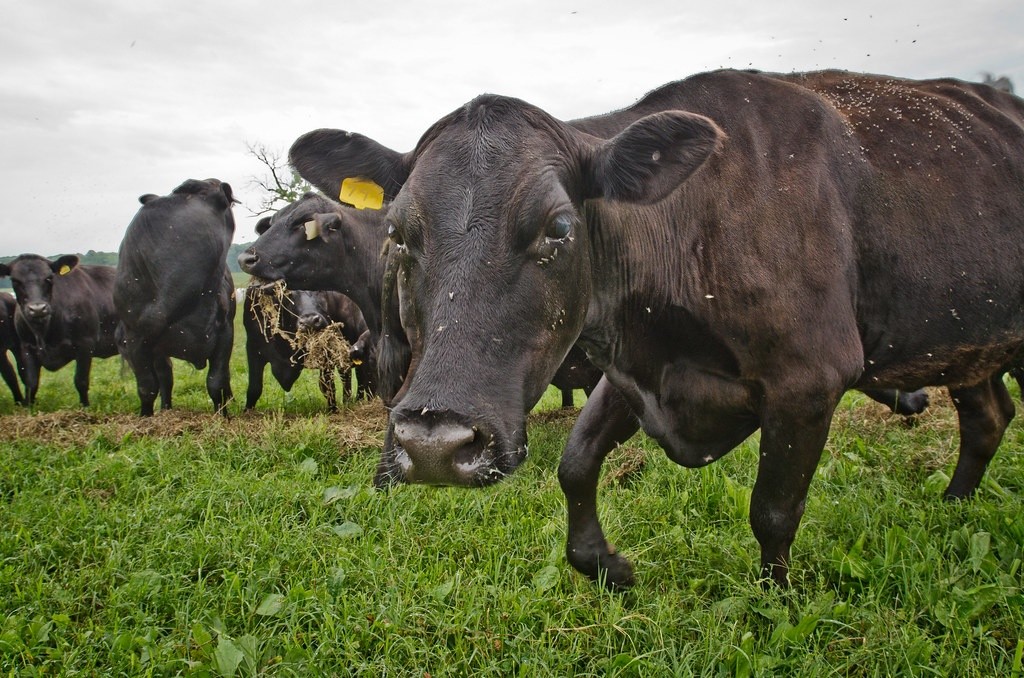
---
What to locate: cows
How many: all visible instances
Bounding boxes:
[0,178,932,491]
[284,67,1024,586]
